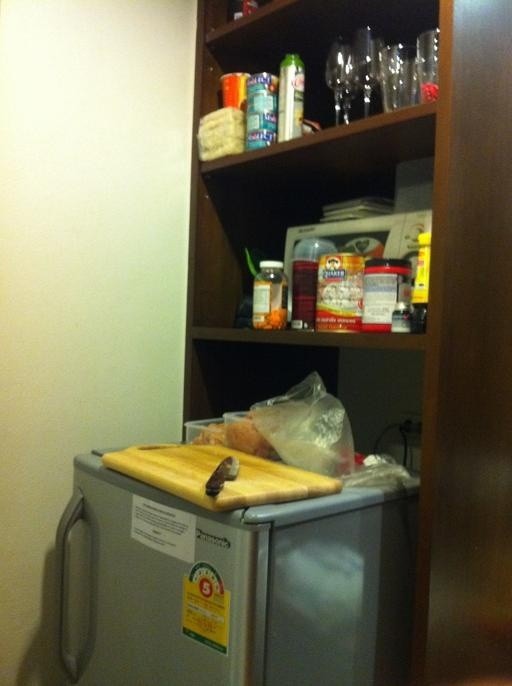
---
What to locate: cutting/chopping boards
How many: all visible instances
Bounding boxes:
[101,444,343,513]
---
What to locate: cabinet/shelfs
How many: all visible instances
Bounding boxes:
[182,0,511,684]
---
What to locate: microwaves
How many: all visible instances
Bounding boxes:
[284,209,432,279]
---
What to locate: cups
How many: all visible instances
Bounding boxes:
[381,43,413,113]
[414,29,440,106]
[219,72,248,110]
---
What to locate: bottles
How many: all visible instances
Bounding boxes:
[411,230,431,305]
[392,301,411,335]
[277,52,306,141]
[251,259,289,334]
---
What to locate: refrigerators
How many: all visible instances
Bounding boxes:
[55,450,419,684]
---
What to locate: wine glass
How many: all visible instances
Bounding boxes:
[324,40,357,127]
[355,27,382,119]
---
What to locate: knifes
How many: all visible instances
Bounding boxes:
[206,454,240,498]
